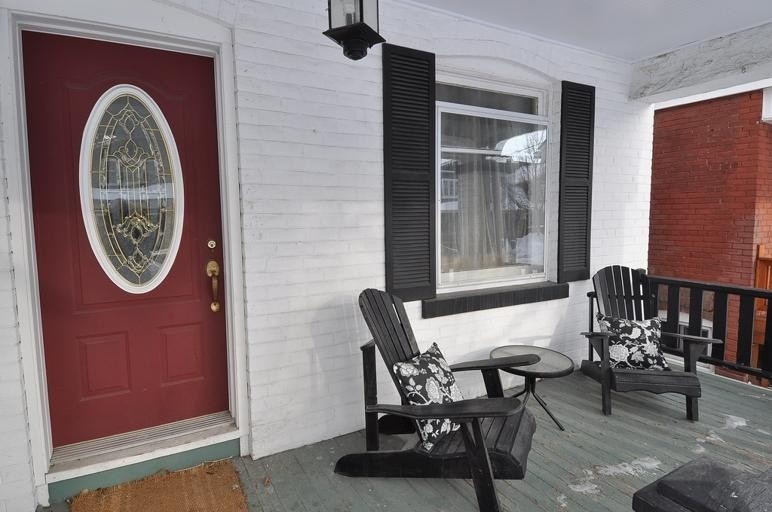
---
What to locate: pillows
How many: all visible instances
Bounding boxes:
[593,309,673,372]
[390,340,465,452]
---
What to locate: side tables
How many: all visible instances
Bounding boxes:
[488,345,575,432]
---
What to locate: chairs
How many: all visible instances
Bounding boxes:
[334,287,541,512]
[580,264,724,421]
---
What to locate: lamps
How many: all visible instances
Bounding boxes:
[322,1,387,62]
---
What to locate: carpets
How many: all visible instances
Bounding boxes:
[65,457,248,511]
